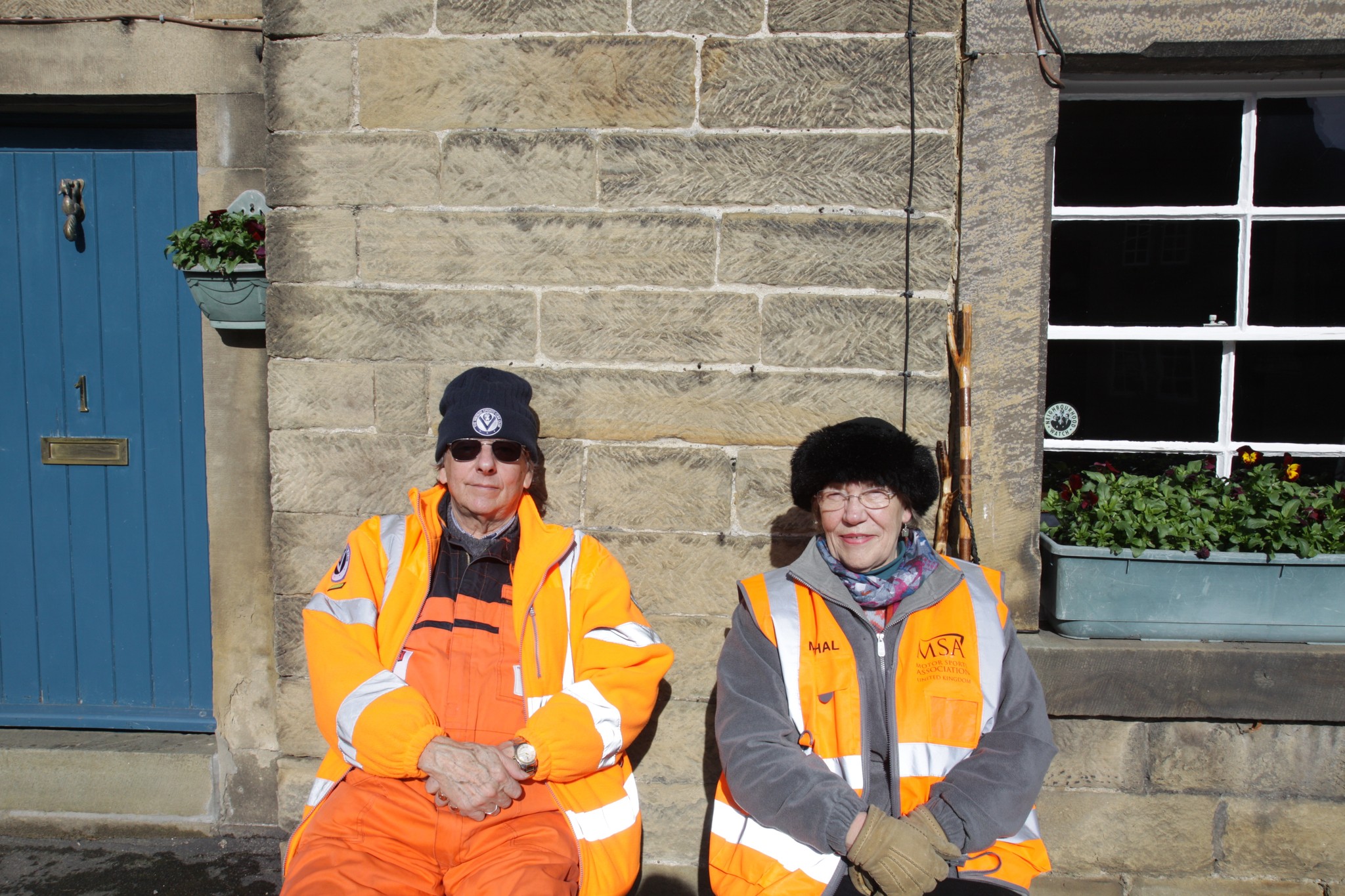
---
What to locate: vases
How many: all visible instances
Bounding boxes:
[171,190,267,331]
[1038,531,1345,645]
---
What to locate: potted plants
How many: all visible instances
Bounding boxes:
[163,207,273,277]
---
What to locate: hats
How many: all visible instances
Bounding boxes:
[790,416,941,516]
[434,366,540,463]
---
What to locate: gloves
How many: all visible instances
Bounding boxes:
[846,803,961,896]
[849,804,949,896]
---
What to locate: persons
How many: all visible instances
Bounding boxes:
[281,366,677,896]
[709,416,1057,896]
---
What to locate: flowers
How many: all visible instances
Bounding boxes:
[1030,445,1345,559]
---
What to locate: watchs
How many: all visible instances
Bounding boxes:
[508,736,537,779]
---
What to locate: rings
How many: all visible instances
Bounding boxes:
[449,801,459,811]
[438,789,449,801]
[484,804,498,814]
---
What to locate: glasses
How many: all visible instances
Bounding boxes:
[448,438,525,463]
[814,487,895,511]
